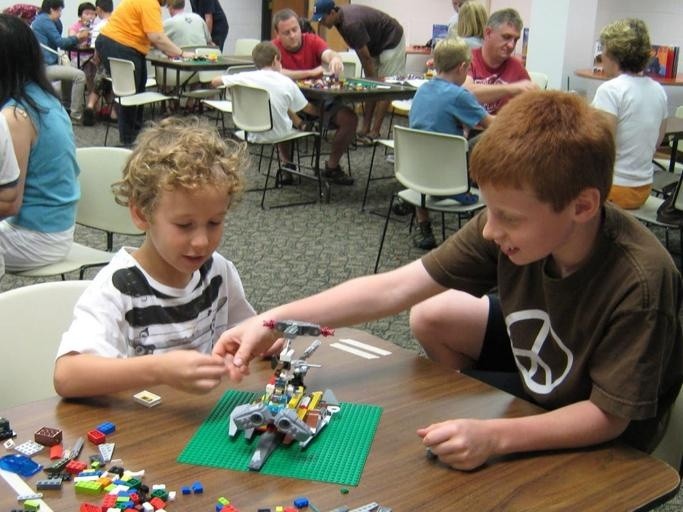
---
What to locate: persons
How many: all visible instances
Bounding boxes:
[391,40,501,249]
[211,42,340,143]
[310,1,406,147]
[587,18,668,210]
[210,89,682,471]
[1,15,81,274]
[52,114,286,402]
[0,0,228,147]
[451,0,492,52]
[269,7,357,183]
[0,114,20,204]
[299,16,315,34]
[462,9,541,130]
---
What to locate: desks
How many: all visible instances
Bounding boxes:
[0,329,680,512]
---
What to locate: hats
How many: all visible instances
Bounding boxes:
[310,0,336,22]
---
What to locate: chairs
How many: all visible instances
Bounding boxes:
[372,126,487,274]
[0,280,94,411]
[8,146,146,280]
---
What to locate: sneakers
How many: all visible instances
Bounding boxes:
[82,107,97,127]
[413,221,436,250]
[279,163,296,185]
[352,134,381,147]
[392,197,416,217]
[325,160,355,186]
[103,117,119,129]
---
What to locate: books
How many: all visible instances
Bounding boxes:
[644,45,679,77]
